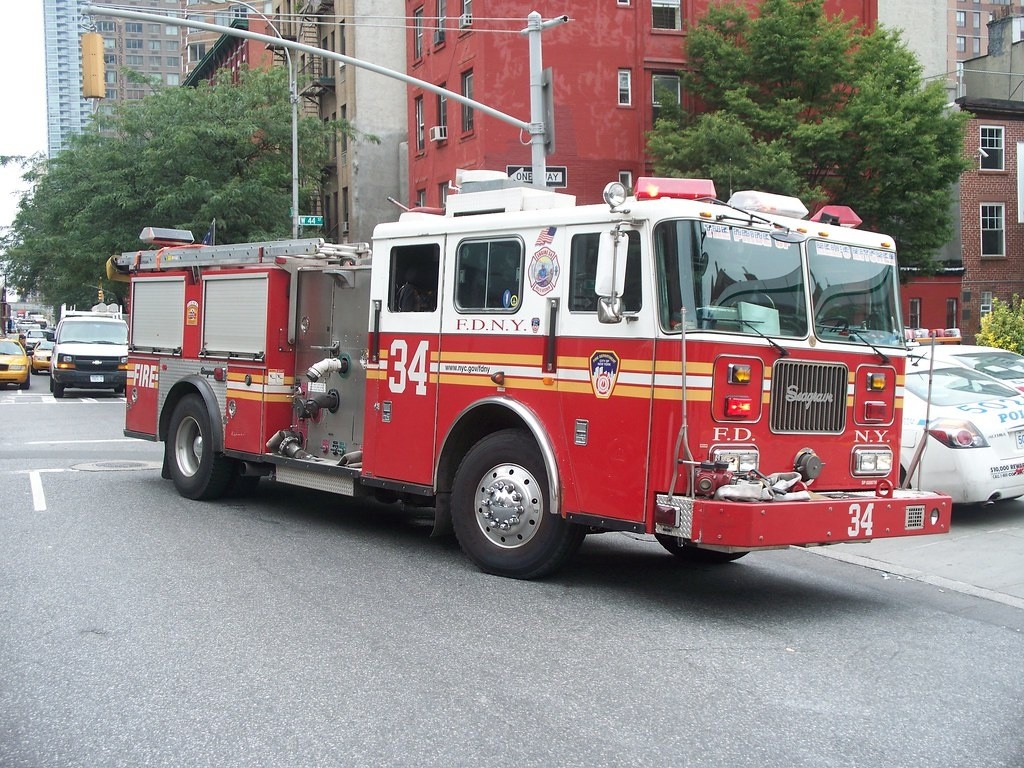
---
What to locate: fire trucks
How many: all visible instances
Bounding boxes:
[102,164,953,581]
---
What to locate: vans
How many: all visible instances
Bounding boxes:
[47,315,130,399]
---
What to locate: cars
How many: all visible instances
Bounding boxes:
[919,341,1024,398]
[902,346,1024,506]
[0,337,31,390]
[13,309,57,374]
